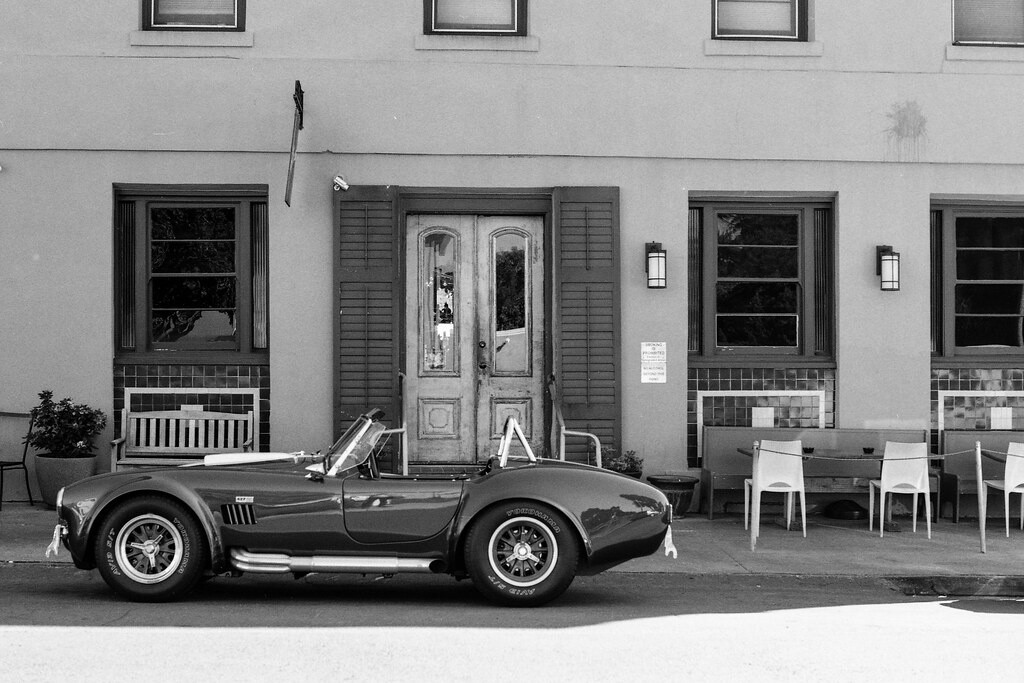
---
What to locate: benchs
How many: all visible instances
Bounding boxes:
[939,429,1024,523]
[701,424,941,523]
[109,387,257,470]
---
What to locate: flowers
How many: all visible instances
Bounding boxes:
[20,388,106,457]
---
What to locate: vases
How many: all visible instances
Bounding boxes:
[34,452,98,510]
[648,474,698,520]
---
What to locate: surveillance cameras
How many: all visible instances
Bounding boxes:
[334,177,350,191]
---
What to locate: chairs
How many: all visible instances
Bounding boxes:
[869,441,932,541]
[744,440,807,538]
[470,457,501,480]
[0,421,35,511]
[979,441,1024,537]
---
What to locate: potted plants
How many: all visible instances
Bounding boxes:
[590,442,642,481]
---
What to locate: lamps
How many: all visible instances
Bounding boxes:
[645,242,667,289]
[876,245,900,292]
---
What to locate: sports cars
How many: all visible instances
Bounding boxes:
[55,407,674,607]
